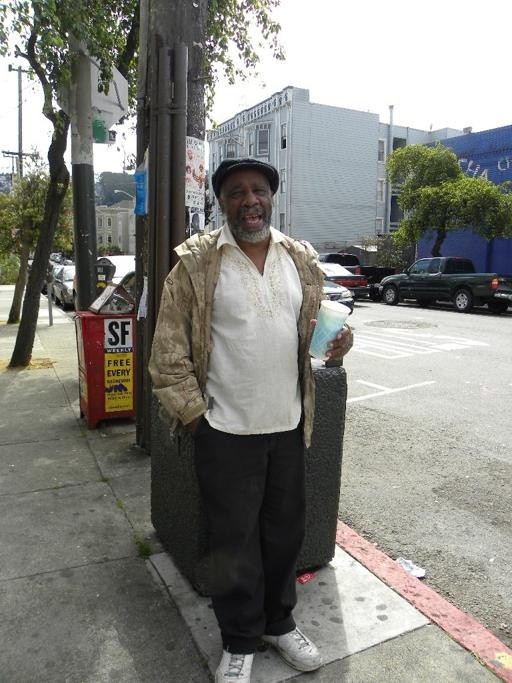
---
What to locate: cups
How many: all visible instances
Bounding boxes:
[307,298,351,361]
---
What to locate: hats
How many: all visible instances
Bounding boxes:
[210,157,280,199]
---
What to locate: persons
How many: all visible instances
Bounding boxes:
[148,156,355,683]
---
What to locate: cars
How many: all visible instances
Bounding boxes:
[49,256,135,311]
[318,263,370,316]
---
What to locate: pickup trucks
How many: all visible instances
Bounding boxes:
[379,257,512,313]
[319,254,399,301]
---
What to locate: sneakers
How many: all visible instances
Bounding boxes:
[260,626,324,673]
[213,648,255,682]
